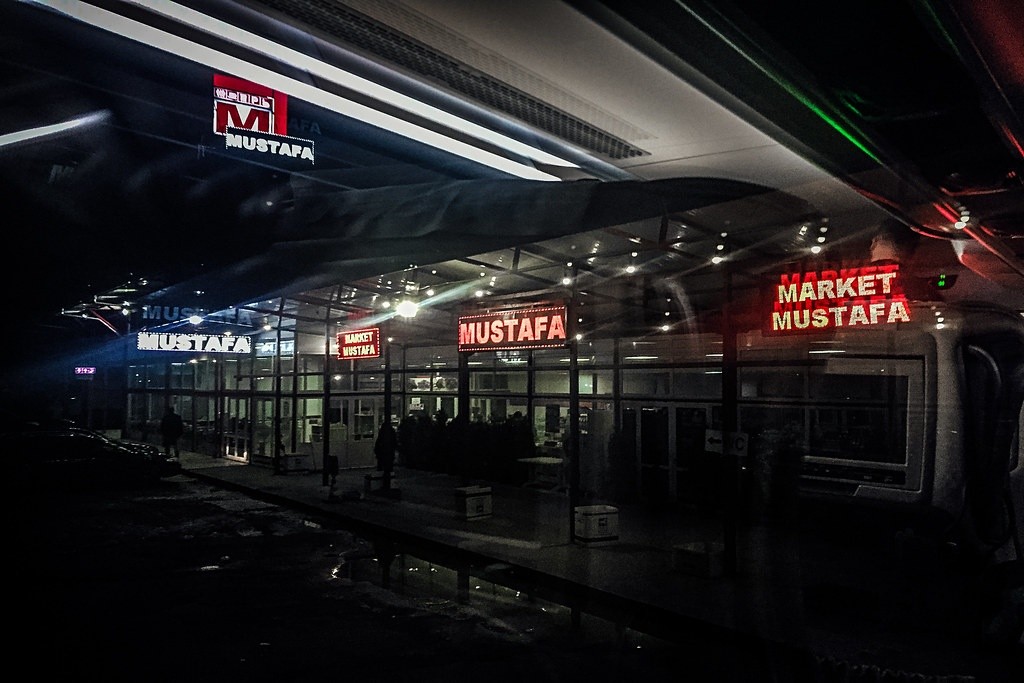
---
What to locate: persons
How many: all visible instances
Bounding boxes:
[160,406,184,456]
[377,419,404,486]
